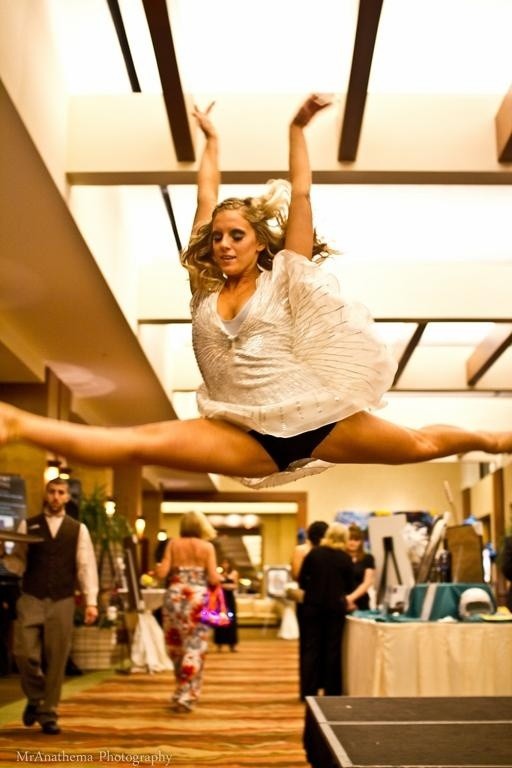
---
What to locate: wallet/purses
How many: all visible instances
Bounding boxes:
[286,587,304,603]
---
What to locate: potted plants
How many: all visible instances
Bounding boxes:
[68,490,132,670]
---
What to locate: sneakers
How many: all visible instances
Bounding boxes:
[172,687,199,712]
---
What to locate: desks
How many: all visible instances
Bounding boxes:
[340,609,512,695]
[302,695,512,768]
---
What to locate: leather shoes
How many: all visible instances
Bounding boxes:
[23,699,61,734]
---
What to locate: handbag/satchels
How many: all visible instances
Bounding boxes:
[200,587,231,628]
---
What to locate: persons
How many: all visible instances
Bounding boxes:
[290,520,328,578]
[345,524,376,611]
[2,477,99,734]
[214,558,239,652]
[0,94,512,489]
[295,521,353,700]
[155,511,221,713]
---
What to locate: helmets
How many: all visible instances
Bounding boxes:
[458,587,494,616]
